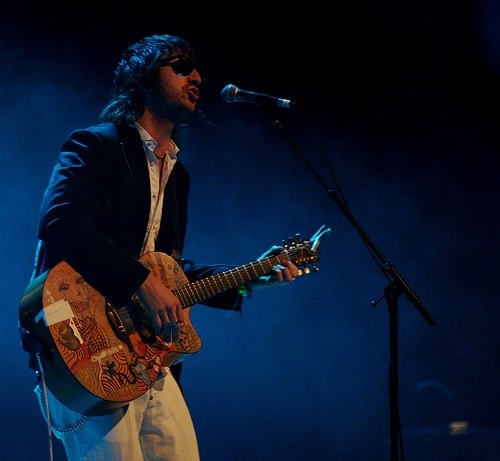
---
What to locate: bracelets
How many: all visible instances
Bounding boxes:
[238,283,248,297]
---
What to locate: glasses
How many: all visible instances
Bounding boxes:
[160,54,208,81]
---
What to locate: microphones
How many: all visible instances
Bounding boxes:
[220,84,296,107]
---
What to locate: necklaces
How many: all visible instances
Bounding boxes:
[154,153,166,160]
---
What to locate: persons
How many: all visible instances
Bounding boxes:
[28,34,298,461]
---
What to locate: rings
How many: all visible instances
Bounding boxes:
[162,322,171,328]
[169,319,178,327]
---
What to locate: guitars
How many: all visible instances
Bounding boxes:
[17,233,323,417]
